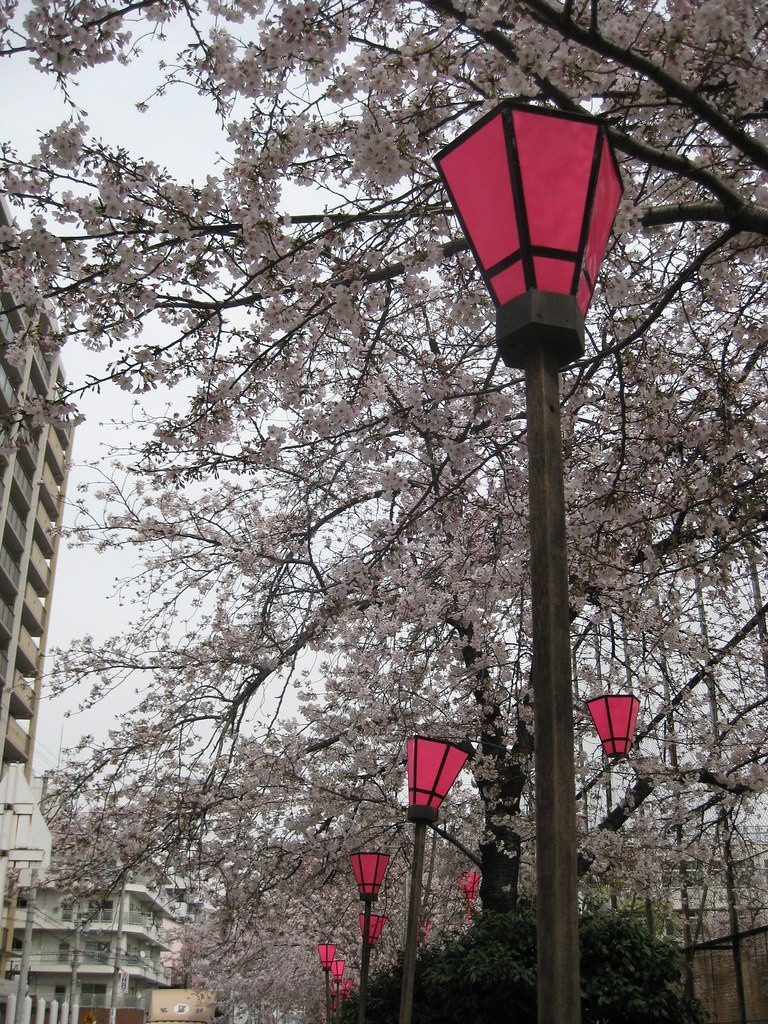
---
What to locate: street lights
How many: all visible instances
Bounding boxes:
[318,943,339,1024]
[397,735,469,1023]
[329,959,346,1024]
[435,96,628,1024]
[350,851,392,1023]
